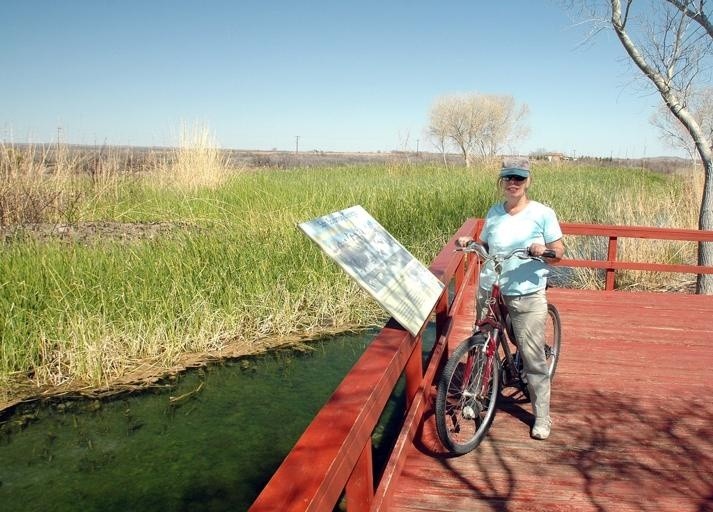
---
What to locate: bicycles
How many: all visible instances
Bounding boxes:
[434,237,560,453]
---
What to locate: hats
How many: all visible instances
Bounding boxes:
[500,157,531,178]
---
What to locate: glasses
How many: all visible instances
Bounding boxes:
[501,175,527,182]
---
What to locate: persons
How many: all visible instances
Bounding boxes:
[454,160,564,438]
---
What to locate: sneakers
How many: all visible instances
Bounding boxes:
[531,414,552,439]
[463,399,487,419]
